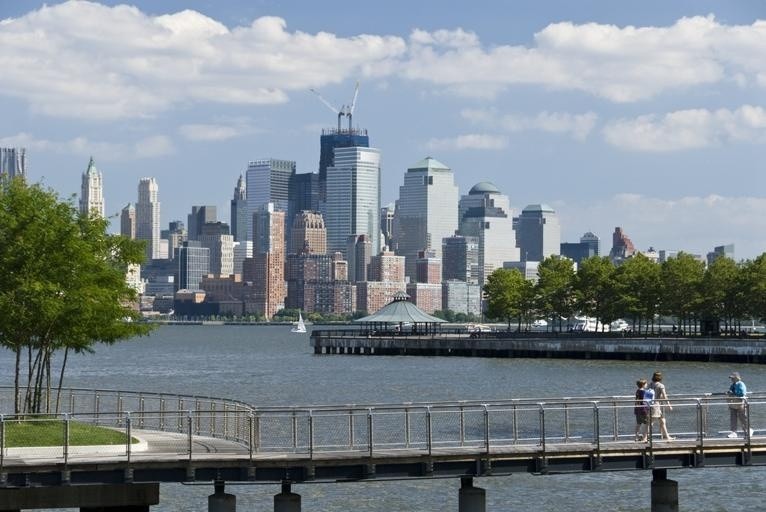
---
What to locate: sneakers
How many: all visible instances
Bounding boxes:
[635,437,650,443]
[747,428,754,438]
[728,432,737,438]
[664,436,677,440]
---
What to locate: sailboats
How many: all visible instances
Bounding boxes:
[290,310,308,334]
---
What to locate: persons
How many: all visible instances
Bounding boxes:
[724,370,755,438]
[641,372,677,441]
[392,326,401,335]
[367,327,377,335]
[634,377,653,442]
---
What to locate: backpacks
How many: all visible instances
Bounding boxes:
[643,389,656,406]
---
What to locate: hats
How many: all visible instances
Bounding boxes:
[729,371,741,378]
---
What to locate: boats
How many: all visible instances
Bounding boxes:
[572,314,631,332]
[461,322,492,332]
[532,318,547,327]
